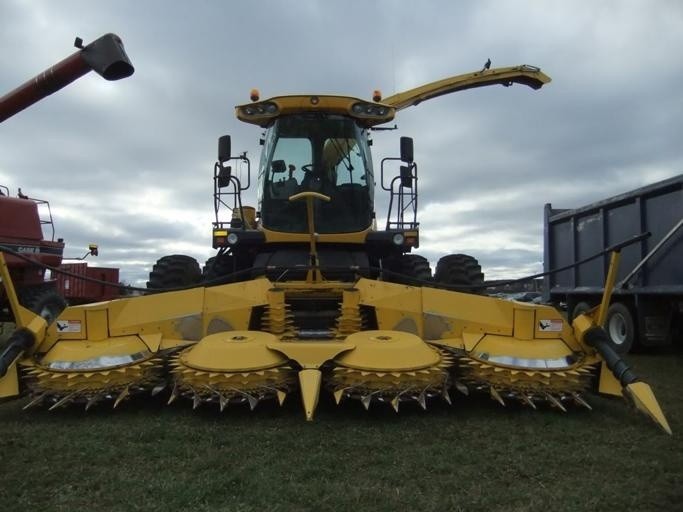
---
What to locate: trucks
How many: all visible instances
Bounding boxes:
[541,173,681,352]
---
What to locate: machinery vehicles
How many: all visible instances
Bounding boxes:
[1,57,676,438]
[1,33,134,325]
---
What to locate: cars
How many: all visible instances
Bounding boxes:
[511,291,568,310]
